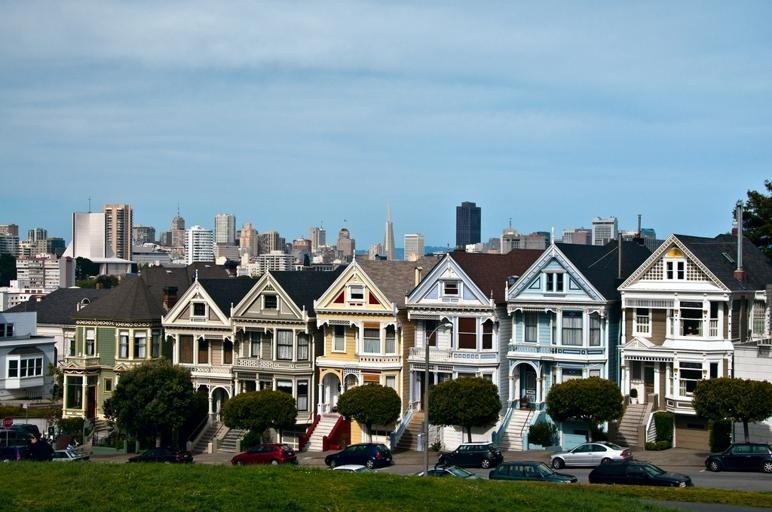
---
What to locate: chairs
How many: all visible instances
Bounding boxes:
[525,467,535,475]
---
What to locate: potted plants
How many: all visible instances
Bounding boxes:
[630,388,639,405]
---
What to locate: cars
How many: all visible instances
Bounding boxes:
[231,444,297,465]
[412,442,503,480]
[0,424,90,462]
[550,441,693,488]
[325,443,392,473]
[705,442,772,473]
[128,447,193,464]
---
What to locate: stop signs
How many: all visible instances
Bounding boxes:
[2,416,14,430]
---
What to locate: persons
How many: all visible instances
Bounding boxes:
[29,432,55,462]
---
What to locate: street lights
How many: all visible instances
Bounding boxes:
[723,353,736,443]
[423,322,453,476]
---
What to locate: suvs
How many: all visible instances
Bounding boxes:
[489,461,578,484]
